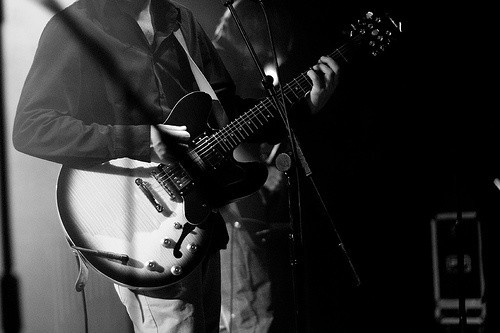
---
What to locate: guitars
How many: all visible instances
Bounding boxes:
[55,5,407,294]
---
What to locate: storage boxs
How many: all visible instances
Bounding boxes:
[430,211,488,333]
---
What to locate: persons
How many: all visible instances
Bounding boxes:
[14,0,342,333]
[217,0,286,333]
